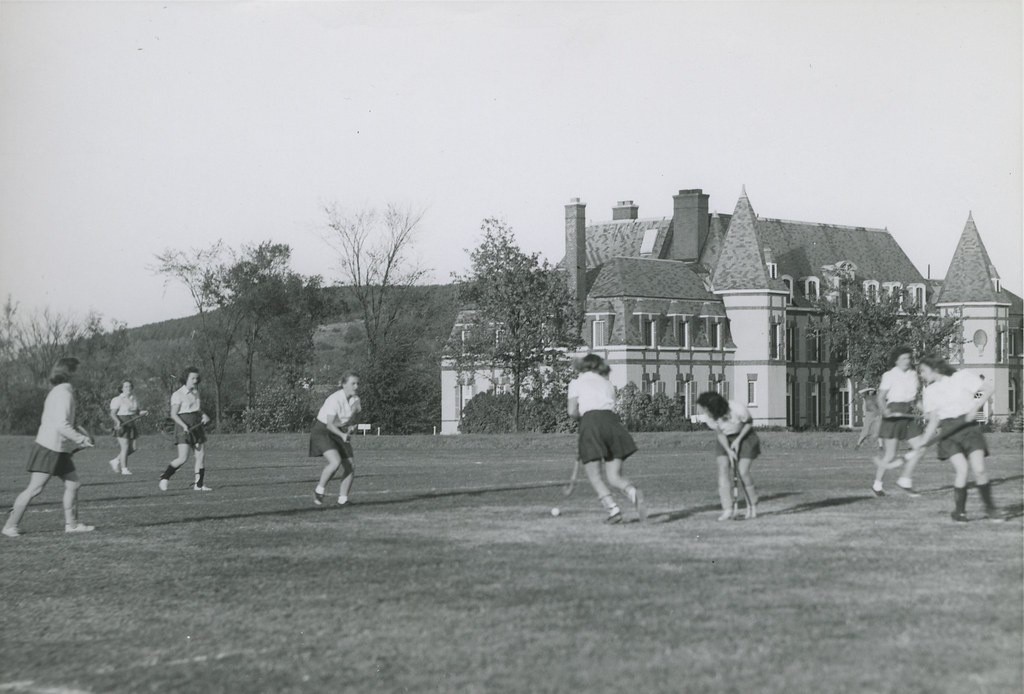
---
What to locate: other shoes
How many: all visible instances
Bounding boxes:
[3,524,20,537]
[159,474,168,491]
[109,459,120,473]
[122,468,132,475]
[313,489,324,505]
[980,506,1004,521]
[871,484,887,500]
[894,480,920,497]
[336,500,356,508]
[194,484,213,491]
[745,503,757,519]
[718,508,738,521]
[65,522,95,533]
[951,512,970,525]
[630,488,645,515]
[605,511,623,526]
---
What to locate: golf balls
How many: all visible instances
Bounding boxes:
[552,507,559,515]
[731,447,746,521]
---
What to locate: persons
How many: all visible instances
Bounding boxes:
[696,392,761,521]
[158,366,212,491]
[308,372,362,506]
[856,386,883,450]
[919,356,1000,522]
[567,354,645,524]
[2,358,95,537]
[872,346,926,498]
[109,380,149,475]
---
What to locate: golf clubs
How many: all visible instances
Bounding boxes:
[100,412,147,433]
[161,421,205,444]
[72,426,94,453]
[347,417,360,440]
[563,454,581,495]
